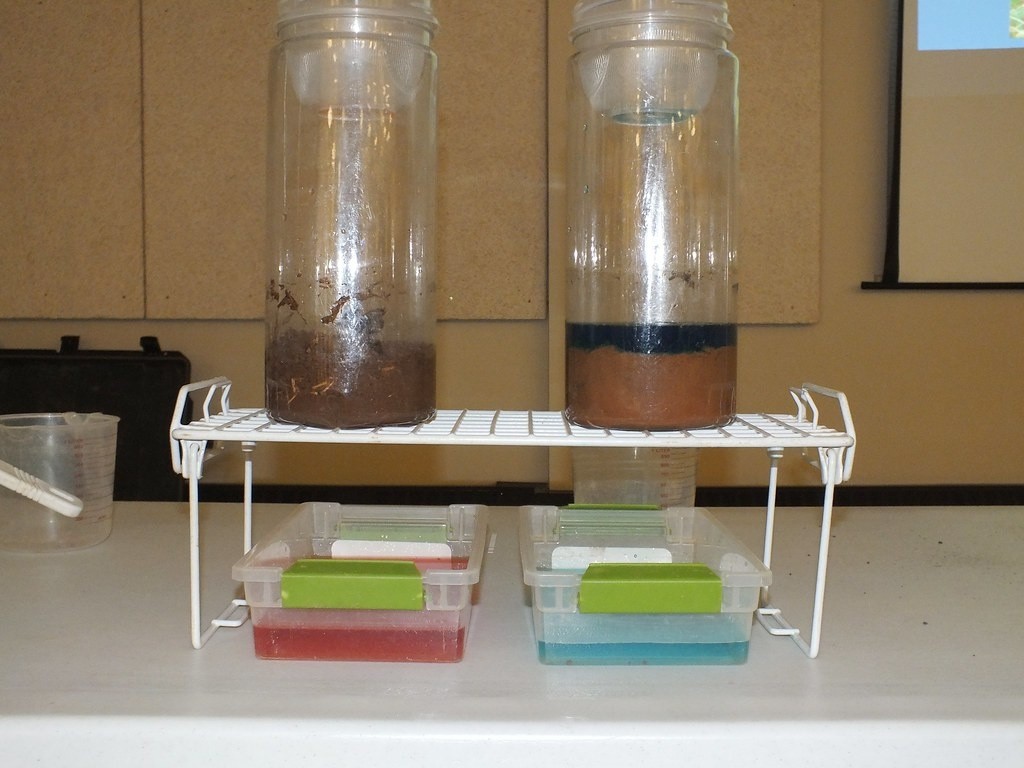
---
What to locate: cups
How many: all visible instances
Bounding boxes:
[0,413,121,552]
[262,0,438,431]
[564,0,740,434]
[572,445,698,508]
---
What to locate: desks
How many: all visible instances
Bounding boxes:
[0,506,1024,768]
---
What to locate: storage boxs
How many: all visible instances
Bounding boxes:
[516,504,775,662]
[229,499,493,663]
[0,336,192,500]
